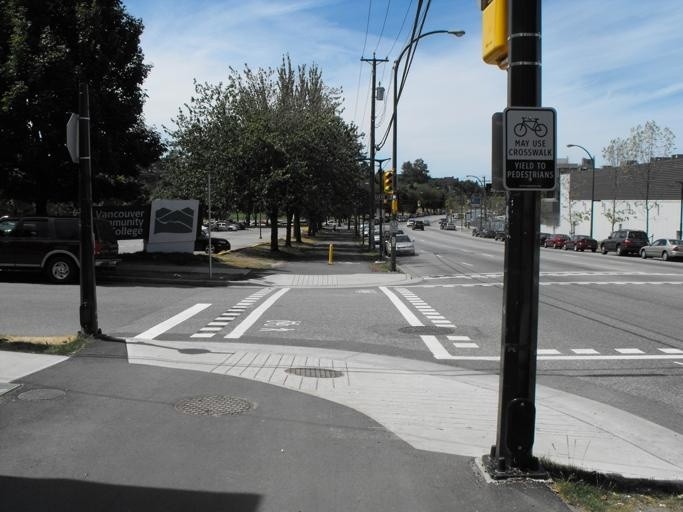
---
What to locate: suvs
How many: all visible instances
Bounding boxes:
[600,228,650,257]
[0,212,121,285]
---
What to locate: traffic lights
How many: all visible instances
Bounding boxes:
[382,172,395,195]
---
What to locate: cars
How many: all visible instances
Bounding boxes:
[440,206,506,231]
[640,239,682,261]
[346,212,435,259]
[201,217,340,232]
[471,227,600,254]
[194,230,232,255]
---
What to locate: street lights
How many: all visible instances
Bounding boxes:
[466,175,489,225]
[565,143,599,241]
[348,156,392,263]
[387,29,467,272]
